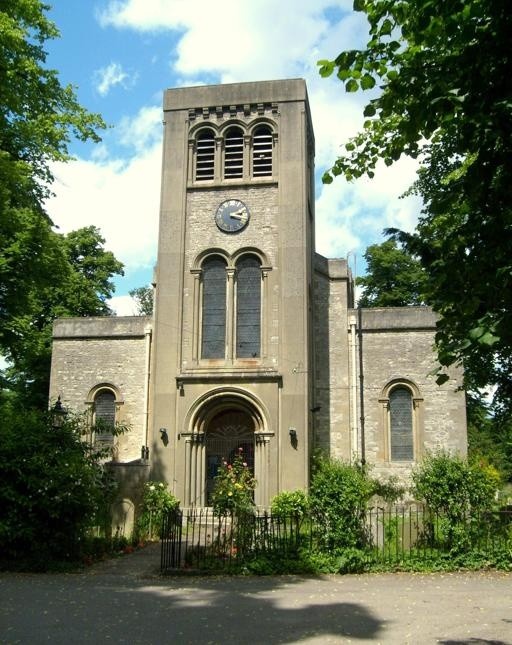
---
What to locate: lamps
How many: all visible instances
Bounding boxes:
[160,429,166,439]
[290,426,296,436]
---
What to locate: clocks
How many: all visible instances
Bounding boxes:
[213,199,250,232]
